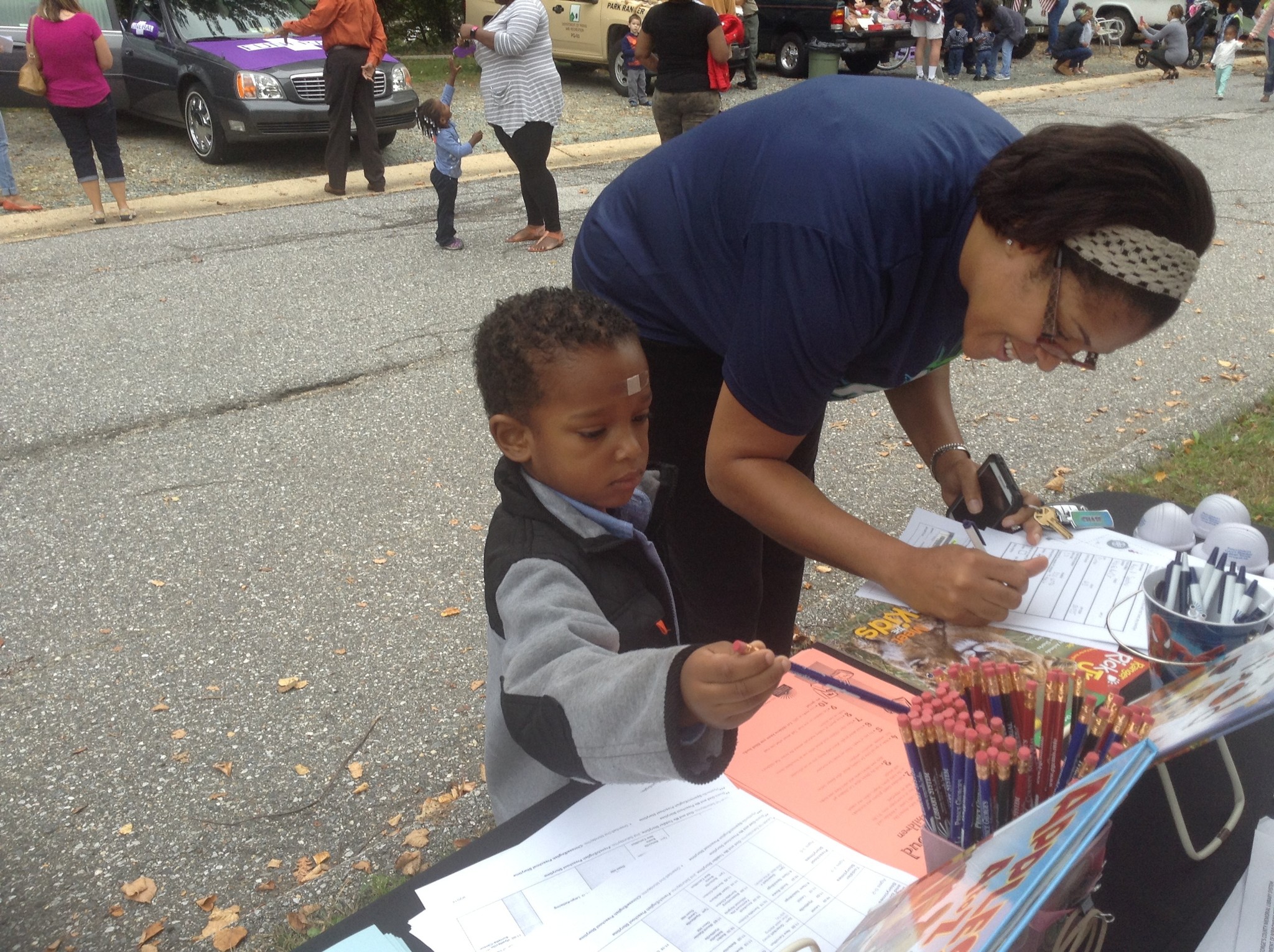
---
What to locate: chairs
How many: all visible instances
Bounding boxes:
[1093,16,1124,55]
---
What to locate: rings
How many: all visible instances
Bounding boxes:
[273,32,274,35]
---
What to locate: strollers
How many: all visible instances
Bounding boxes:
[1135,6,1217,70]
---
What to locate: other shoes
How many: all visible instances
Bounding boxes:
[966,64,981,75]
[942,65,948,73]
[89,211,106,225]
[629,101,652,107]
[367,183,385,192]
[439,238,464,250]
[119,207,136,221]
[993,73,1010,81]
[1053,64,1074,76]
[737,81,758,90]
[1072,67,1088,75]
[0,194,42,211]
[948,74,958,80]
[324,183,346,196]
[973,72,992,81]
[1260,94,1270,102]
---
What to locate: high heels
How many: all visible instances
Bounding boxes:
[1159,68,1179,80]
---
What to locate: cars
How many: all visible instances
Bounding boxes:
[0,0,420,166]
[754,1,922,79]
[1022,0,1186,47]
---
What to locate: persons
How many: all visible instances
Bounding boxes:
[573,75,1214,656]
[25,0,136,223]
[635,0,731,146]
[0,43,43,211]
[734,0,760,90]
[908,0,1274,105]
[621,14,652,106]
[473,286,792,827]
[456,0,564,251]
[415,55,483,249]
[262,0,388,196]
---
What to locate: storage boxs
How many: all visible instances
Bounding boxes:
[844,20,883,32]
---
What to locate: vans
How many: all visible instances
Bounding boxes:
[464,0,751,98]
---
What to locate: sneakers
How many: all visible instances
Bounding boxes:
[915,74,927,81]
[926,76,944,85]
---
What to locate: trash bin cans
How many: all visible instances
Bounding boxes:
[806,41,845,82]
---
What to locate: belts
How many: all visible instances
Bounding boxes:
[326,44,349,56]
[744,11,757,19]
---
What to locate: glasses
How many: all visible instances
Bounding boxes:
[1036,245,1100,372]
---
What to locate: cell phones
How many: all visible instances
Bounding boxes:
[453,43,476,59]
[1140,16,1143,26]
[945,453,1022,529]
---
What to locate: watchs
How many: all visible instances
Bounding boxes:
[470,26,479,39]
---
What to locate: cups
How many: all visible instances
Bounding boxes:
[1142,566,1274,690]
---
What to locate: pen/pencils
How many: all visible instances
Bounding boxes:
[1198,614,1207,621]
[1202,552,1228,612]
[1217,561,1237,624]
[1187,605,1199,618]
[962,519,1009,587]
[1180,551,1190,616]
[1160,550,1181,612]
[1230,565,1246,624]
[1199,547,1219,599]
[1242,597,1274,624]
[1231,580,1258,624]
[896,655,1156,854]
[1188,567,1203,615]
[731,640,911,713]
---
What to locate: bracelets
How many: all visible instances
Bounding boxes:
[930,443,970,482]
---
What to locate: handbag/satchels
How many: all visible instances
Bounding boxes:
[917,5,941,24]
[18,41,47,97]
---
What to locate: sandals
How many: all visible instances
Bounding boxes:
[504,225,545,242]
[527,231,564,252]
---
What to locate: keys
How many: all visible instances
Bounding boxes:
[1032,505,1074,540]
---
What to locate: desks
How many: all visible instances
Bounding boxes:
[285,492,1274,952]
